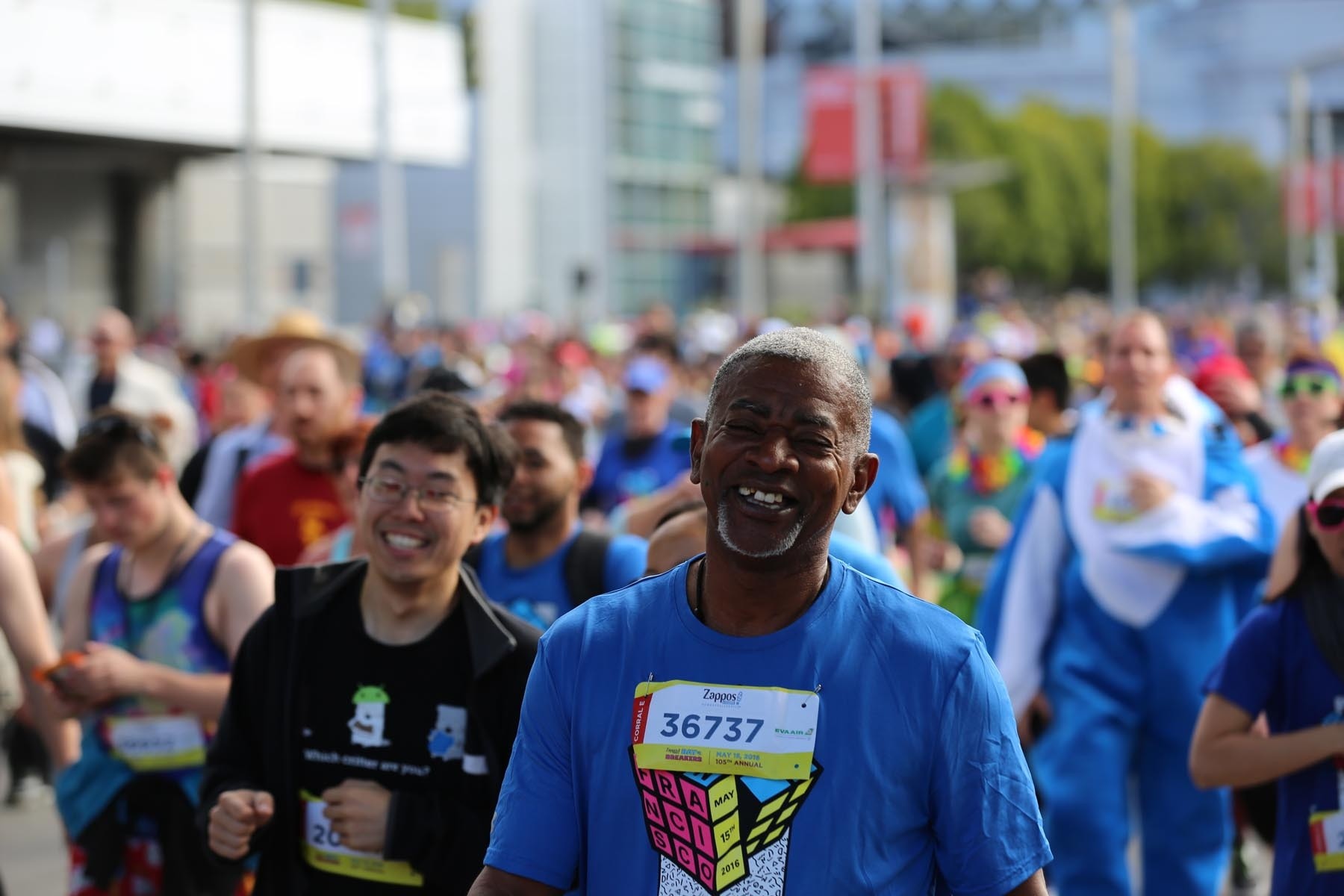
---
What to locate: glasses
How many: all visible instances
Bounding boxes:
[1280,383,1327,398]
[357,472,481,514]
[1,341,27,367]
[1305,501,1344,531]
[974,392,1024,407]
[89,481,150,512]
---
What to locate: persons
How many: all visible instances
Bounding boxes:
[2,295,1344,896]
[641,503,711,588]
[193,390,544,896]
[1189,427,1343,896]
[442,398,653,631]
[970,309,1280,896]
[42,414,276,896]
[461,328,1053,896]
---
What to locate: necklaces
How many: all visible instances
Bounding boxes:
[688,550,831,636]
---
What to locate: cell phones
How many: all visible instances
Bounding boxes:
[36,654,120,716]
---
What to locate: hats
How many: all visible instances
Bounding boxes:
[621,357,667,393]
[1286,352,1338,376]
[555,341,590,368]
[232,310,359,383]
[88,307,132,342]
[1309,429,1344,505]
